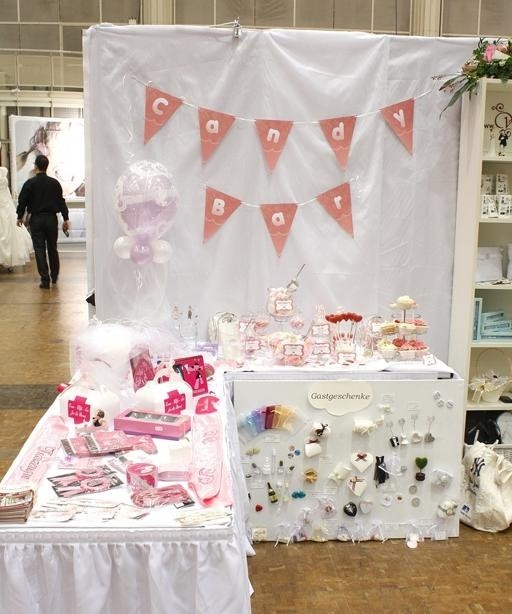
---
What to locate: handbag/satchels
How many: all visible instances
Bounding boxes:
[459,441,511,534]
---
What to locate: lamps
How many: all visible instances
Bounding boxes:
[233,14,242,39]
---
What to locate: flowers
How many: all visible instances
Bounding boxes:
[431,34,511,121]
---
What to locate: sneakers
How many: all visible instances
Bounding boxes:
[39,284,50,289]
[52,278,56,284]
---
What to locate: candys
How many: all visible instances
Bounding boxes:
[325,311,362,339]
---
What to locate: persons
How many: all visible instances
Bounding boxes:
[16,154,71,289]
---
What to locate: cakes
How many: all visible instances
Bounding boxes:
[377,296,430,359]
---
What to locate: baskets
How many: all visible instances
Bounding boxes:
[464,443,511,464]
[474,348,511,403]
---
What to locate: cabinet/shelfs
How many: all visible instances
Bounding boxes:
[223,331,467,541]
[447,64,512,466]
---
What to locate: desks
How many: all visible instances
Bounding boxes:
[2,341,256,613]
